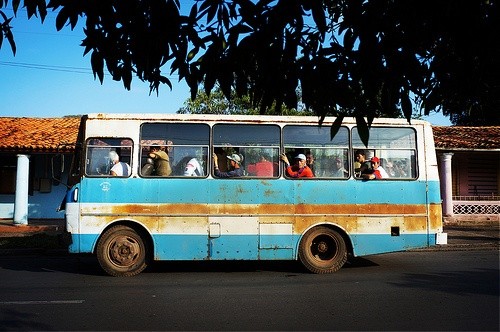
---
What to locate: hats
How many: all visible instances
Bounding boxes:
[227,154,241,164]
[109,151,119,160]
[369,157,380,162]
[295,154,306,161]
[336,157,342,163]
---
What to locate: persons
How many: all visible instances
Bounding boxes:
[109,151,130,176]
[355,150,389,179]
[149,143,172,176]
[247,153,278,177]
[212,152,244,177]
[280,153,320,178]
[330,156,348,177]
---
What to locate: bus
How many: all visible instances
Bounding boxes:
[51,113,448,278]
